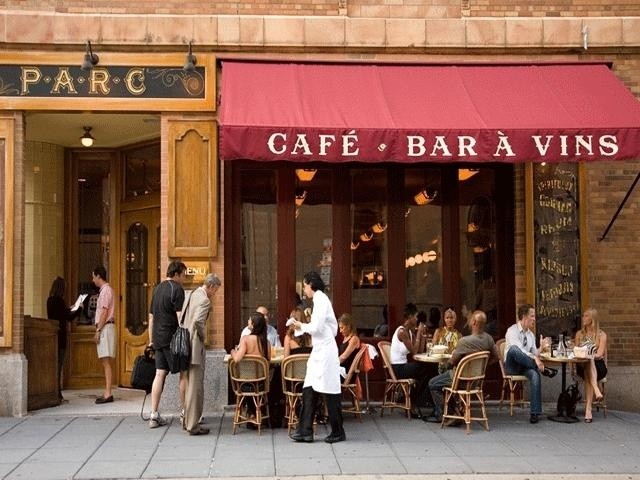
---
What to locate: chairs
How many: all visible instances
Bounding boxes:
[496,339,530,417]
[281,354,317,436]
[321,346,366,422]
[230,354,269,436]
[570,339,607,418]
[440,350,491,434]
[378,340,416,421]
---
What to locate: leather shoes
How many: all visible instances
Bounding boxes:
[289,432,313,442]
[324,435,346,442]
[541,367,558,378]
[95,395,113,404]
[530,413,538,423]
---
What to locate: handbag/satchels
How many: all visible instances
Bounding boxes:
[130,345,155,392]
[169,290,190,375]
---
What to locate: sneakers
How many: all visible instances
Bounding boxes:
[443,414,460,426]
[179,412,204,424]
[150,416,166,427]
[189,428,210,435]
[60,399,68,404]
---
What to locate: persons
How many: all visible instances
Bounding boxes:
[289,272,345,443]
[338,314,360,387]
[503,304,558,424]
[575,308,608,423]
[46,276,84,404]
[284,309,313,395]
[389,303,427,418]
[92,266,117,404]
[230,312,271,428]
[240,307,282,348]
[429,311,500,426]
[432,309,463,356]
[181,273,221,435]
[146,262,188,427]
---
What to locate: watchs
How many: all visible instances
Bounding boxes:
[96,328,101,332]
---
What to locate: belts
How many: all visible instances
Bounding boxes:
[95,321,114,328]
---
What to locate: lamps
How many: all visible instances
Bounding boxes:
[79,127,94,147]
[80,40,98,71]
[182,40,196,73]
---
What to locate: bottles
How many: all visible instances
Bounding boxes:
[557,335,565,359]
[427,340,433,356]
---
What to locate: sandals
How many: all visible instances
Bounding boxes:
[585,414,592,423]
[596,394,604,401]
